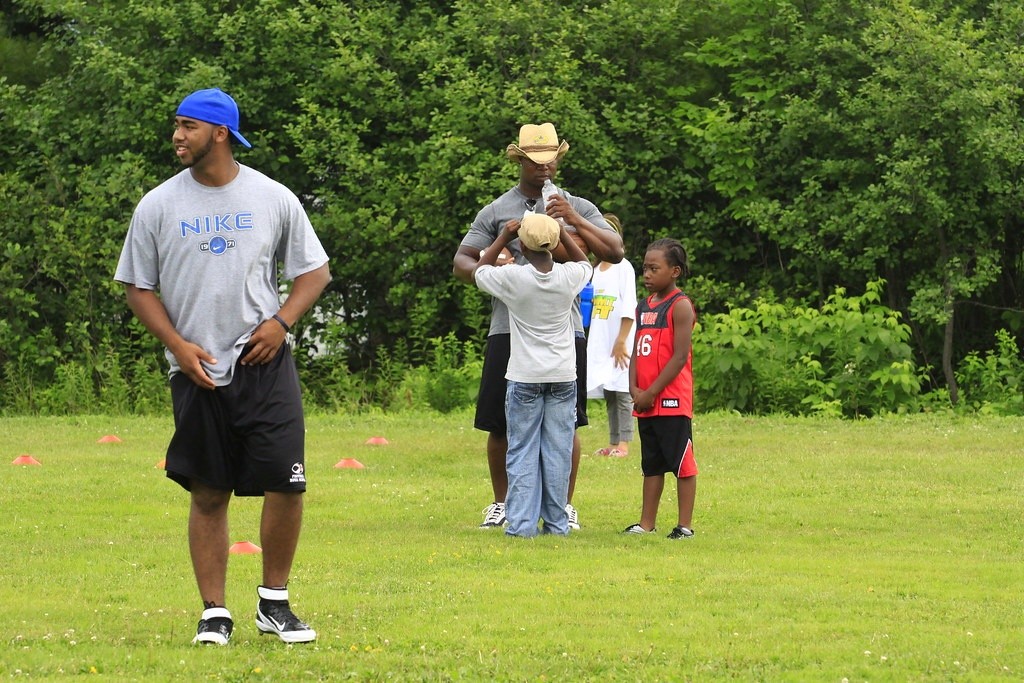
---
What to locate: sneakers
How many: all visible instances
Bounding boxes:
[563,504,580,531]
[193,605,234,646]
[254,583,316,644]
[478,501,509,529]
[666,525,694,540]
[621,523,656,536]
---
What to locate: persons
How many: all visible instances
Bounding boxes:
[114,87,334,646]
[471,214,595,537]
[586,213,637,458]
[615,237,699,539]
[452,122,626,530]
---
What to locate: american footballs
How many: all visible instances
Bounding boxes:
[551,230,590,263]
[479,246,514,265]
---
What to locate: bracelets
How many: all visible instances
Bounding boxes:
[272,315,290,332]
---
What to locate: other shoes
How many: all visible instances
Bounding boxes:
[593,448,613,457]
[608,449,628,457]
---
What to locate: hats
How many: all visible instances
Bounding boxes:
[516,213,560,256]
[177,86,253,153]
[505,121,569,166]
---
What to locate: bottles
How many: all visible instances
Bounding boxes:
[541,179,564,225]
